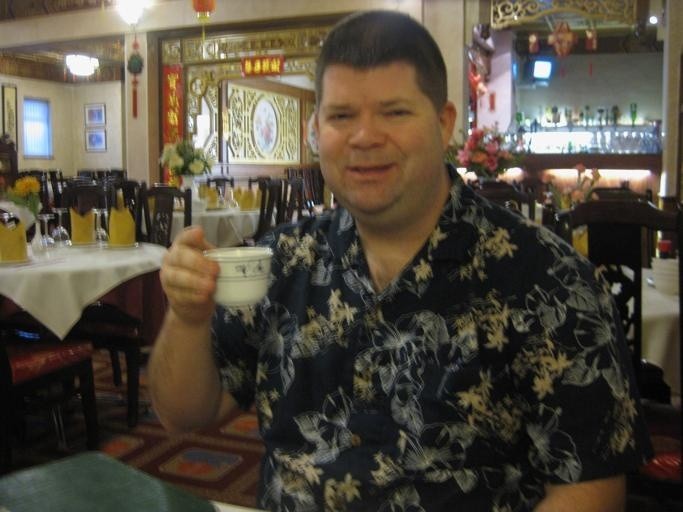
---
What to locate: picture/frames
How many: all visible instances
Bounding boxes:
[1,83,18,150]
[83,102,107,127]
[85,128,107,151]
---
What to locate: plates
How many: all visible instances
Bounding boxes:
[0,258,36,270]
[104,243,142,251]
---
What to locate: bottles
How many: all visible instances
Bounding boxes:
[514,103,639,128]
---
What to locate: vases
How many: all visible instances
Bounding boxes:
[477,173,497,186]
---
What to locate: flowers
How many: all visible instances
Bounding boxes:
[442,120,532,180]
[154,138,215,179]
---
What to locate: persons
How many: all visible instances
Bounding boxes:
[146,8,655,512]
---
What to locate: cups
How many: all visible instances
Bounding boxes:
[202,245,274,312]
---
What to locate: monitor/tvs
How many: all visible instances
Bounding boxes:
[524,56,554,81]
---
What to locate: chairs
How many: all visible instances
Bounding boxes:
[2,168,193,250]
[477,181,681,396]
[204,168,325,240]
[0,301,147,459]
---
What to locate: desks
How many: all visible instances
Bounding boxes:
[171,210,269,243]
[2,240,170,340]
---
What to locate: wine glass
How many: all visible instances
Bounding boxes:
[217,185,239,210]
[30,204,108,267]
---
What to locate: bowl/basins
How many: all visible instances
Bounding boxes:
[191,199,206,212]
[650,257,679,296]
